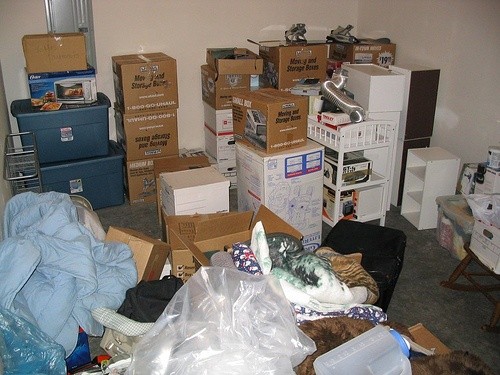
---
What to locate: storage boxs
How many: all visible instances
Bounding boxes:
[0,32,500,375]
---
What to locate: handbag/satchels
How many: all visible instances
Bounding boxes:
[117,275,184,322]
[100,327,145,363]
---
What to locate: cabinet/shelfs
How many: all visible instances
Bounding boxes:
[307,116,398,227]
[400,147,460,231]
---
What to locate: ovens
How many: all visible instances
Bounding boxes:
[53,78,97,105]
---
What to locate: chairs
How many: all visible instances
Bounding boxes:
[440,241,500,334]
